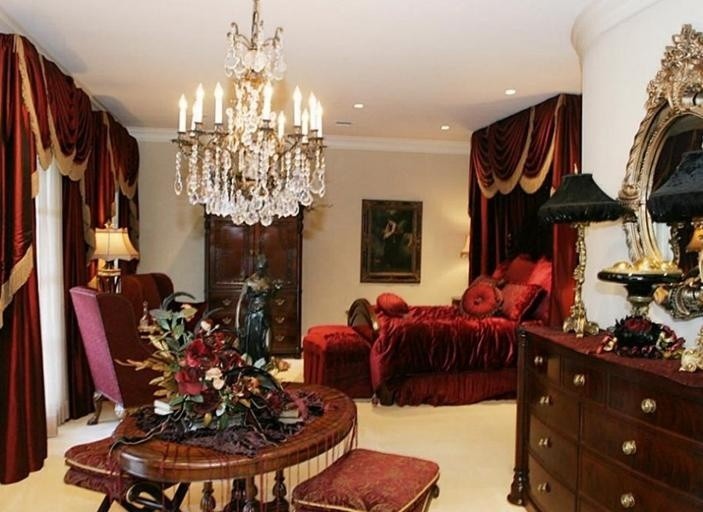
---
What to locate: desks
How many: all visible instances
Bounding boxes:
[110,381,358,512]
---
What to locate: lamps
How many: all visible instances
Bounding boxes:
[170,1,327,227]
[460,234,470,258]
[538,171,624,338]
[645,147,703,371]
[91,225,140,293]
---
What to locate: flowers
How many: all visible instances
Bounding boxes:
[113,289,288,431]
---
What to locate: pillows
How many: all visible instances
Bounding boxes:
[461,254,554,322]
[376,291,412,318]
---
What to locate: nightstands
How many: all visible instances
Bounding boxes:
[450,296,463,308]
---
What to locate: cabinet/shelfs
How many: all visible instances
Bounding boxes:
[505,316,703,511]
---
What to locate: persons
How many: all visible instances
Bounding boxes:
[230,254,287,359]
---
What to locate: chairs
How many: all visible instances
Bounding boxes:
[70,285,167,427]
[130,274,208,343]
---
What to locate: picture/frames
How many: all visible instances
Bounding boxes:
[362,197,424,286]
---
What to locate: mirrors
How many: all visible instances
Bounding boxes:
[613,21,703,322]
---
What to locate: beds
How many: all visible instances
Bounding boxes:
[347,94,581,407]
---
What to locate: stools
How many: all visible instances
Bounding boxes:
[62,437,179,512]
[291,446,440,512]
[301,323,373,401]
